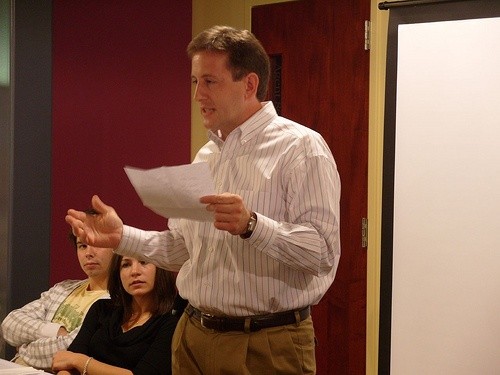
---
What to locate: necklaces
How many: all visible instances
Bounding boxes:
[127,313,150,328]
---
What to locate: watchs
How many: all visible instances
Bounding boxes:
[240,211,257,240]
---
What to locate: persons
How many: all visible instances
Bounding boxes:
[1,208,114,372]
[65,25,341,375]
[51,254,189,375]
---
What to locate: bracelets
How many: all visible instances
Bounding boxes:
[83,357,93,374]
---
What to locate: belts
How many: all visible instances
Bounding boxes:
[184,303,311,332]
